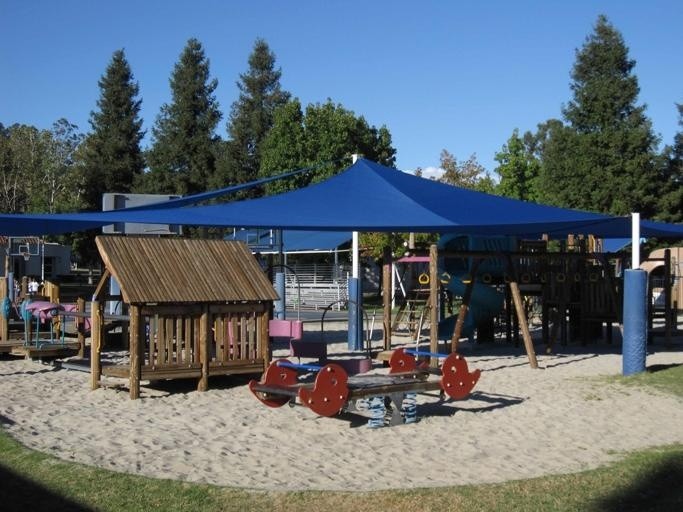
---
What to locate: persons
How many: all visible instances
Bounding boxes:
[12,276,40,297]
[371,244,402,273]
